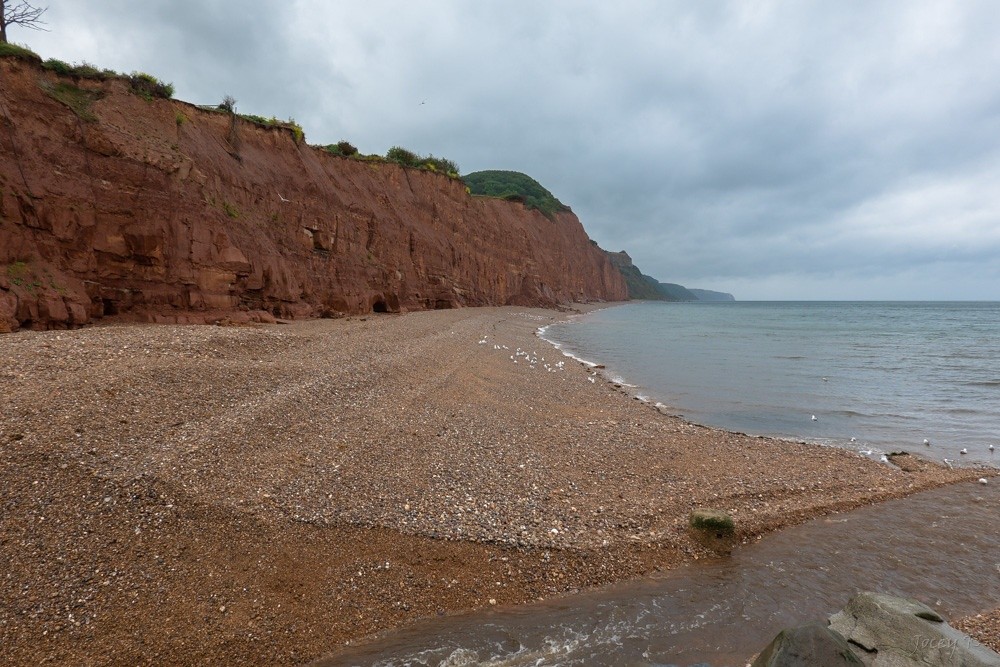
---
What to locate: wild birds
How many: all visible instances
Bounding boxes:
[811,376,994,485]
[478,336,596,384]
